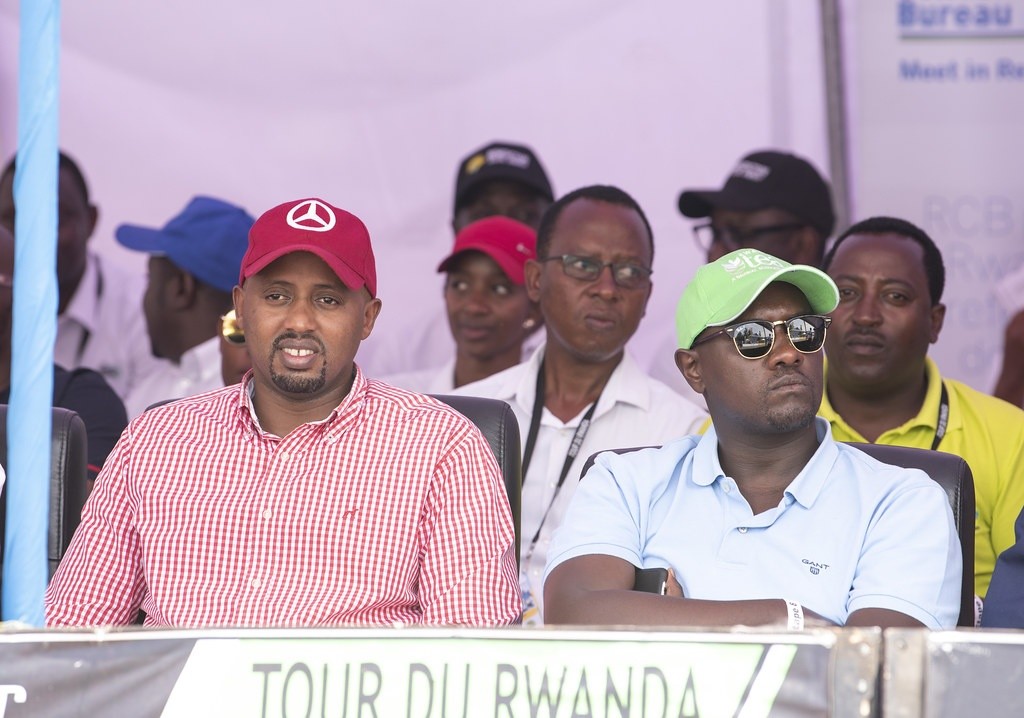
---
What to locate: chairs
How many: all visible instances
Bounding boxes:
[0,405,88,623]
[580,441,976,635]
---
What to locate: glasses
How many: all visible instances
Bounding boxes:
[691,313,834,359]
[693,223,808,251]
[544,253,654,288]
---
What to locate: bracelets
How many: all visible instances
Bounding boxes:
[974,595,983,627]
[783,598,804,629]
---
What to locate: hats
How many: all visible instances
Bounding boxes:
[676,247,839,349]
[456,142,553,208]
[437,215,537,285]
[240,197,377,299]
[678,151,832,236]
[115,195,256,290]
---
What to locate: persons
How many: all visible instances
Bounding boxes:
[44,198,522,628]
[449,184,712,625]
[384,217,538,391]
[0,148,166,392]
[979,505,1024,629]
[114,195,256,420]
[704,217,1024,627]
[808,328,812,342]
[993,311,1024,410]
[0,227,128,497]
[450,142,556,363]
[543,249,964,630]
[145,306,252,412]
[679,150,838,269]
[768,333,772,346]
